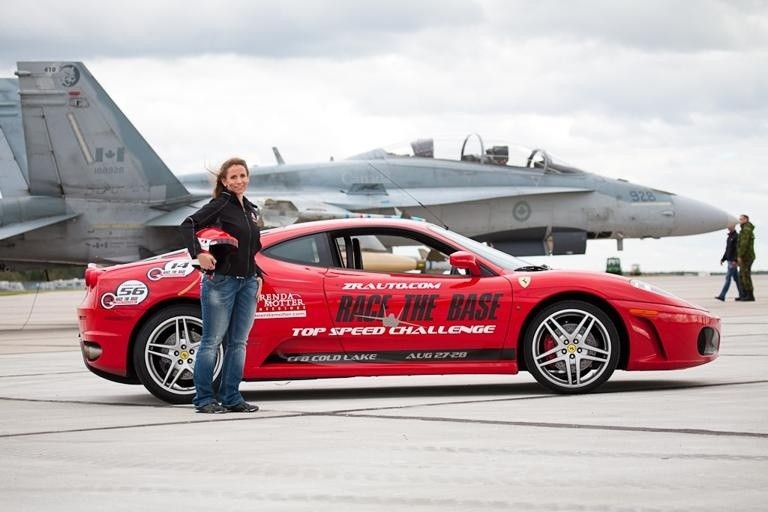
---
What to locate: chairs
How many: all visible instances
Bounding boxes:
[342,233,367,273]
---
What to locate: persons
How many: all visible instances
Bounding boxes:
[734,214,757,302]
[177,155,266,416]
[713,224,741,302]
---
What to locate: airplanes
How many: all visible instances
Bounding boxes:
[0,58,737,288]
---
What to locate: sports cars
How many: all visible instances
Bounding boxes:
[77,215,729,399]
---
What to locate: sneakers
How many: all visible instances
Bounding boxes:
[197,403,227,413]
[221,401,258,412]
[716,296,754,301]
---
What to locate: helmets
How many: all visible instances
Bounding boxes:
[187,227,239,275]
